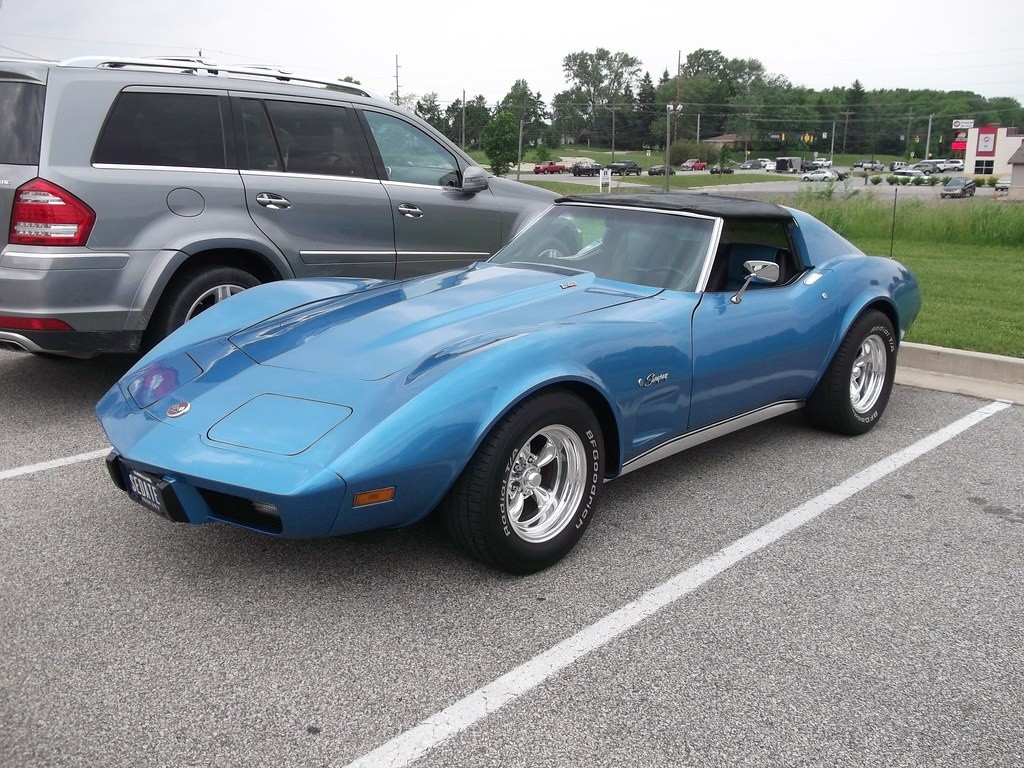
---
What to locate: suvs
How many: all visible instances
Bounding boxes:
[889,161,908,171]
[893,158,965,186]
[940,176,976,199]
[0,51,584,369]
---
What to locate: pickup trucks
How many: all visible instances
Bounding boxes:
[681,158,708,172]
[862,160,885,171]
[533,161,565,175]
[606,160,642,177]
[813,158,832,168]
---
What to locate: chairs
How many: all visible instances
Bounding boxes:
[287,118,345,175]
[708,242,790,292]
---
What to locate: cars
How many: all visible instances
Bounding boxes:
[828,169,848,182]
[648,165,677,176]
[994,176,1012,191]
[801,161,818,173]
[765,162,776,172]
[710,165,734,174]
[854,159,866,168]
[802,169,839,182]
[757,158,771,168]
[740,159,761,170]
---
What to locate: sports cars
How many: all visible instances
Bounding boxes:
[96,190,921,577]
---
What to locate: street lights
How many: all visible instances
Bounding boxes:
[663,104,684,192]
[516,117,535,182]
